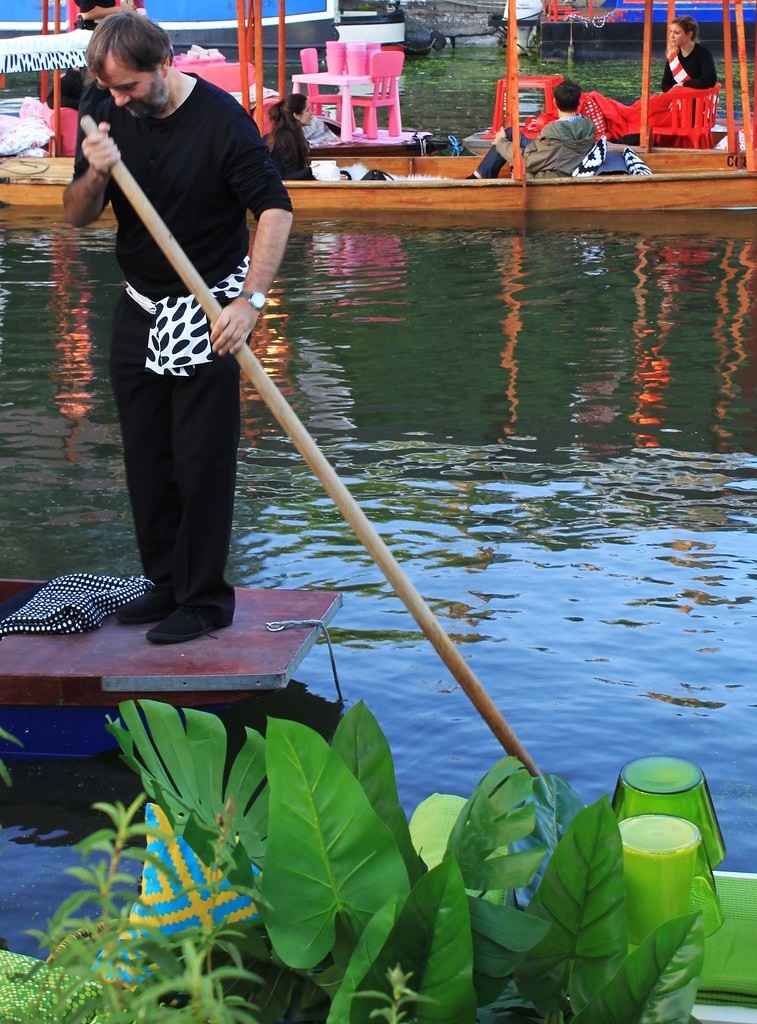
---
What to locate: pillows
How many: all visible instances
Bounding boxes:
[0,949,138,1024]
[0,97,56,157]
[622,145,653,176]
[409,793,509,909]
[573,134,608,177]
[90,803,264,1004]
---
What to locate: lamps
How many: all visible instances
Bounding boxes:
[617,813,725,946]
[611,756,727,870]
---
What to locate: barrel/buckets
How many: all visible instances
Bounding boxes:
[310,160,337,181]
[46,107,79,157]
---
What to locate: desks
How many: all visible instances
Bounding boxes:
[493,75,564,132]
[292,72,402,142]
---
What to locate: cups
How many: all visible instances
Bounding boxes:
[616,812,725,949]
[612,756,726,870]
[180,45,224,61]
[326,41,383,77]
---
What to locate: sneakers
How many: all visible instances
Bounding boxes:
[115,590,179,623]
[146,606,232,642]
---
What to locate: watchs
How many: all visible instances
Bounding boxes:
[238,290,266,311]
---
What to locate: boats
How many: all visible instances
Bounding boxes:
[0,575,353,765]
[0,0,757,219]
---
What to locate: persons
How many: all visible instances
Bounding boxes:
[660,16,716,92]
[47,0,296,644]
[466,81,592,179]
[257,94,352,181]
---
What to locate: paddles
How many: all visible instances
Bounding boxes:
[75,108,541,777]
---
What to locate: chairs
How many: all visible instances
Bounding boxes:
[351,51,405,140]
[205,63,256,93]
[650,83,722,149]
[51,107,78,156]
[300,48,342,124]
